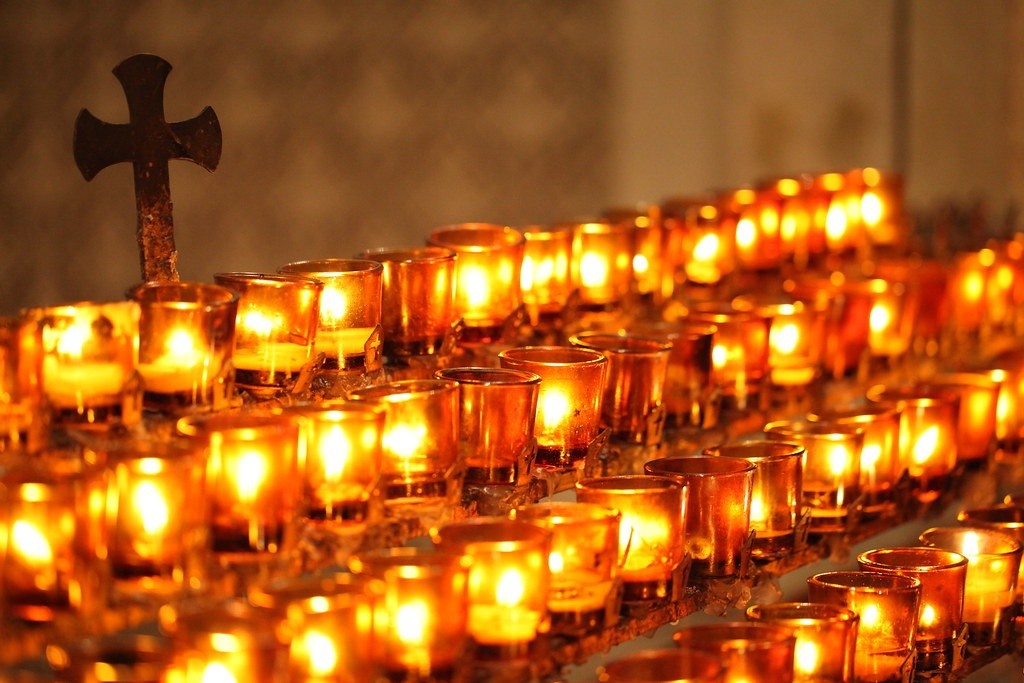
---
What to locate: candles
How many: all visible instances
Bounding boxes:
[0,165,1024,683]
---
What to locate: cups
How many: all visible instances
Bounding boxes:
[0,168,1024,683]
